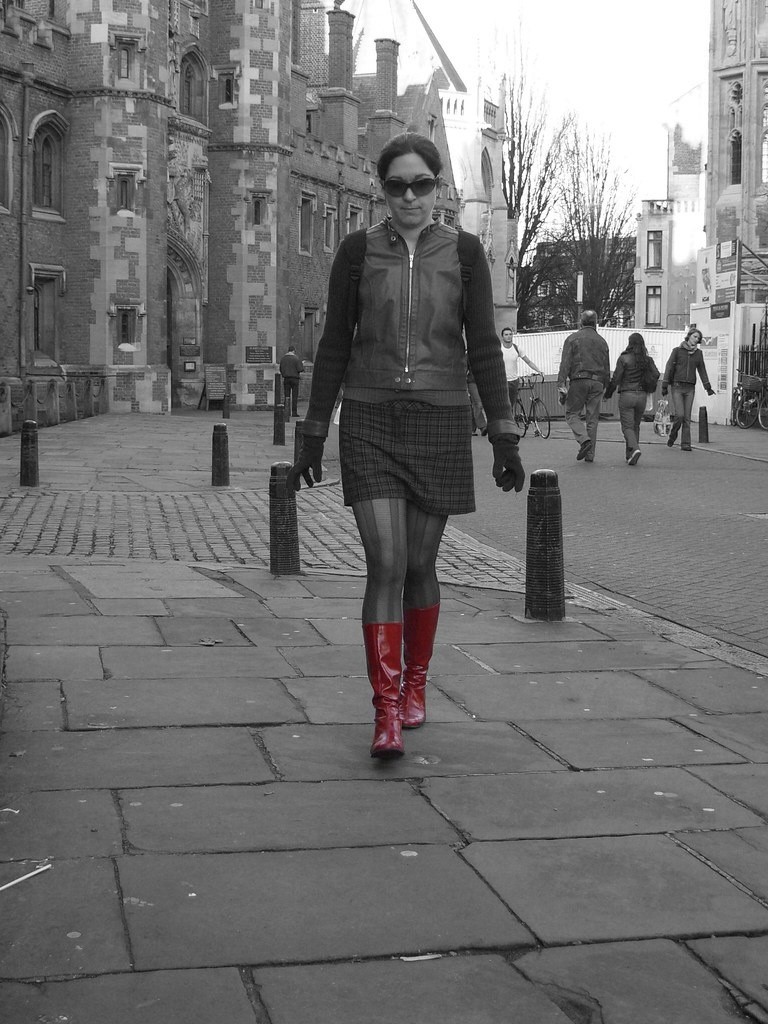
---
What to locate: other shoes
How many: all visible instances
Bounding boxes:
[628,450,641,465]
[585,457,593,462]
[667,438,675,447]
[292,415,300,417]
[481,426,488,436]
[471,431,478,436]
[576,441,593,460]
[681,445,691,451]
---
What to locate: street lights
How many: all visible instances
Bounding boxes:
[575,270,585,329]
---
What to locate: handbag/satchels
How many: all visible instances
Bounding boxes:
[653,395,671,437]
[641,356,660,393]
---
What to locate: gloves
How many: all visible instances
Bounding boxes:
[489,433,525,493]
[604,391,613,400]
[707,388,716,396]
[285,435,326,491]
[662,387,668,396]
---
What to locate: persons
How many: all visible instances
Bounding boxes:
[466,351,488,436]
[662,328,716,451]
[557,310,611,462]
[284,133,524,755]
[280,346,304,417]
[602,333,649,464]
[501,327,545,420]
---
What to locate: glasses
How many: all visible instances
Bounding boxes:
[381,175,440,197]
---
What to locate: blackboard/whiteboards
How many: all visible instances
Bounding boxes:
[204,363,228,399]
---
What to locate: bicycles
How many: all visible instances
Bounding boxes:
[731,369,768,430]
[510,373,551,439]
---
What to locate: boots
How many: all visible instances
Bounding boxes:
[362,622,405,757]
[398,603,440,729]
